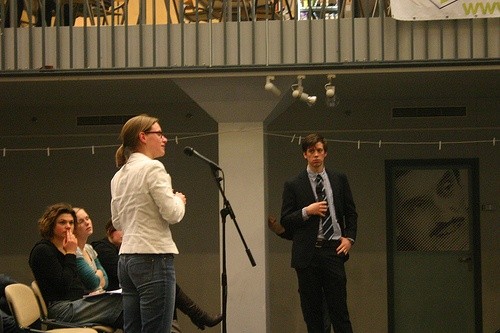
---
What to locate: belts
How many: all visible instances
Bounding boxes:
[312,241,341,247]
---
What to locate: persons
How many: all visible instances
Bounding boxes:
[268,133,360,333]
[110,112,187,333]
[0,0,114,27]
[91,218,222,331]
[72,206,109,289]
[391,169,470,253]
[29,202,124,331]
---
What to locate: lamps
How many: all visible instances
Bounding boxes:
[264,74,341,108]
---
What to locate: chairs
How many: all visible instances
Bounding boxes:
[5,280,117,333]
[78,0,216,26]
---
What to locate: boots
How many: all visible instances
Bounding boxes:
[175,289,224,330]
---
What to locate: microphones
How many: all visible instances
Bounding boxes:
[183,146,222,172]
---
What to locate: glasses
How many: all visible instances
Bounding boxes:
[145,131,164,136]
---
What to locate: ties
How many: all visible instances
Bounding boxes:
[315,174,335,240]
